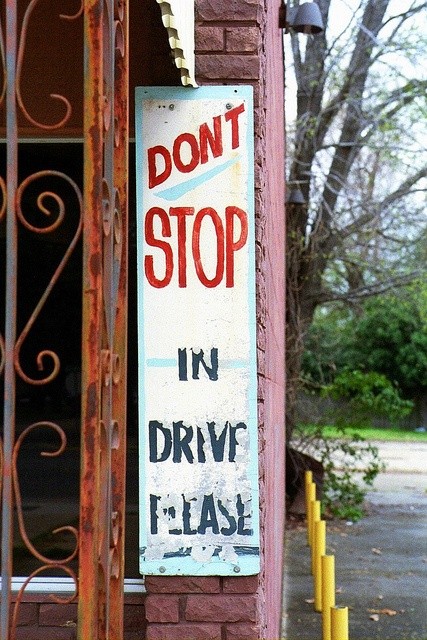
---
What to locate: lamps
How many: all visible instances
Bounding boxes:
[286,0,323,41]
